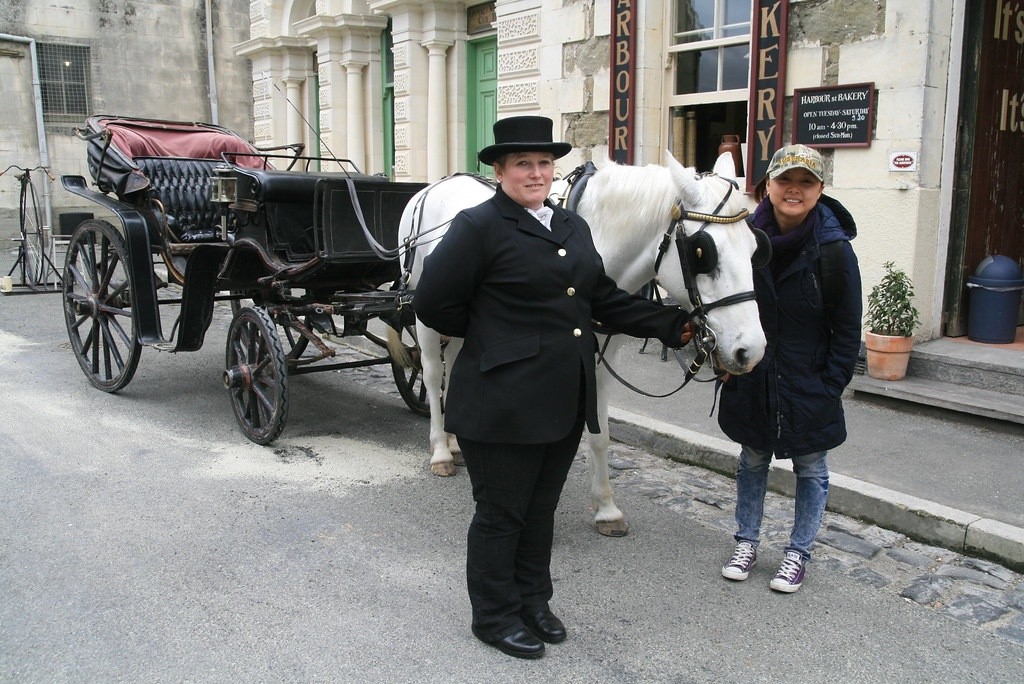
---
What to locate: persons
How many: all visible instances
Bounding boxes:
[412,116,694,659]
[715,144,863,592]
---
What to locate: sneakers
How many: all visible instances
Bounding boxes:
[769,550,806,592]
[722,540,757,580]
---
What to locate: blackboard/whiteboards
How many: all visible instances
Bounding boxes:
[790,81,875,149]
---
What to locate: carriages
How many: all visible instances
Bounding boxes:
[61,113,768,536]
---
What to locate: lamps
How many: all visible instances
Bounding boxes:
[209,163,237,241]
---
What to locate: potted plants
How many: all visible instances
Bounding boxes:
[863,259,923,382]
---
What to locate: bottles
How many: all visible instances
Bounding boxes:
[719,135,740,176]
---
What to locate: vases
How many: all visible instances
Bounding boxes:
[718,134,742,177]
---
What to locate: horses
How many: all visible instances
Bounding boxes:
[398,150,767,537]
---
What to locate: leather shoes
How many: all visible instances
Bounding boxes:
[492,608,566,659]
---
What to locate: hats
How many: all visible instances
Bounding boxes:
[766,144,824,183]
[479,116,572,166]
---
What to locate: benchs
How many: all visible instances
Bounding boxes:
[132,155,232,257]
[221,151,390,258]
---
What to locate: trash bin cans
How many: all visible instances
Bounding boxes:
[968,255,1024,343]
